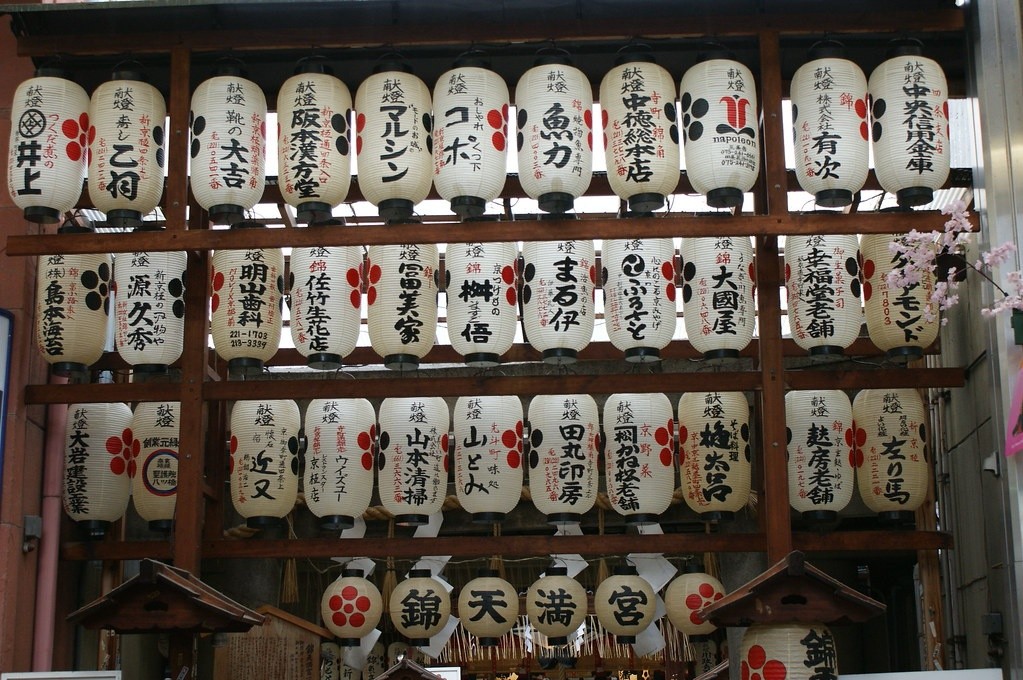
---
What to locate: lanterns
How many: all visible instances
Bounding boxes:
[8,54,979,680]
